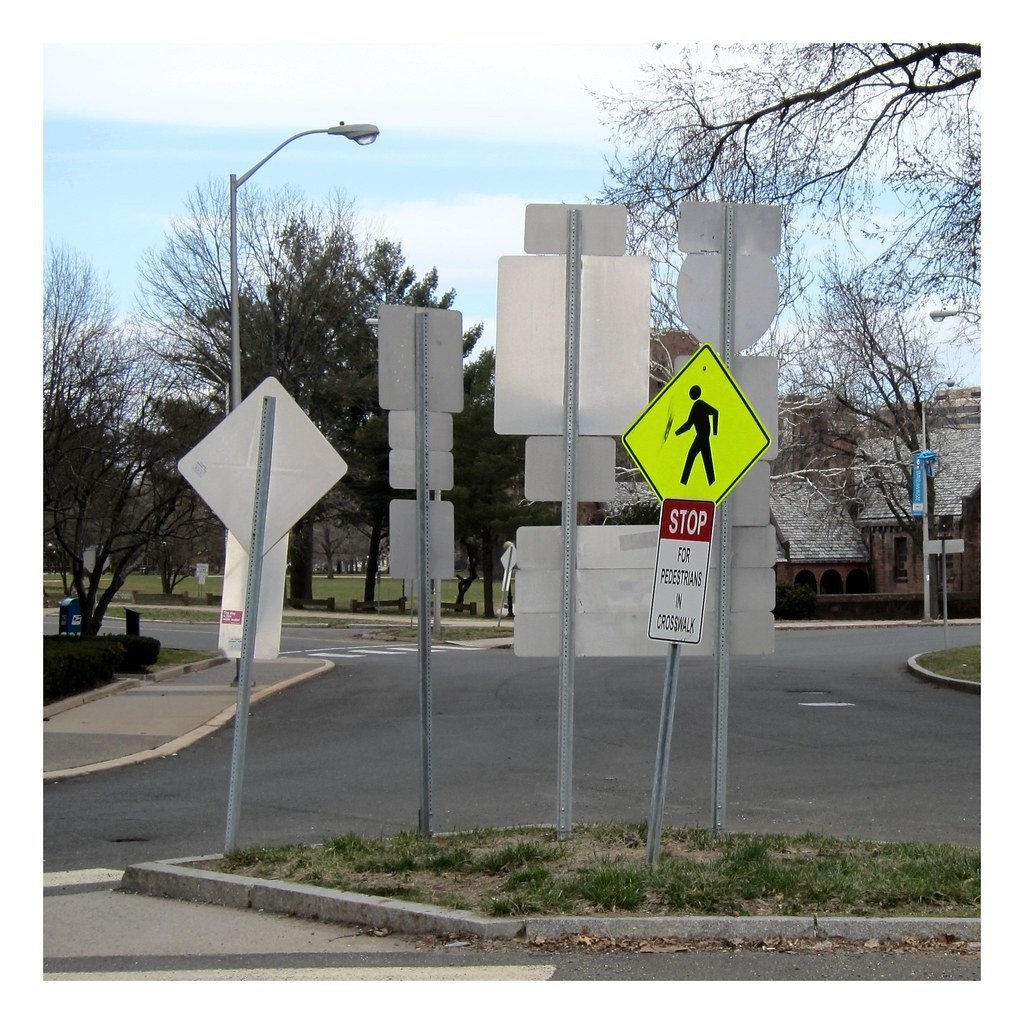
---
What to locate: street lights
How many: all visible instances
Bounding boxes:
[233,119,380,688]
[921,377,956,621]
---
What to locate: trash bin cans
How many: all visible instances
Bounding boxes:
[56,597,83,636]
